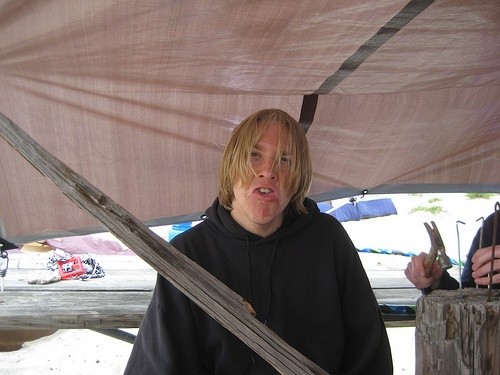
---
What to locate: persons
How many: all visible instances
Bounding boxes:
[404,208,500,295]
[126,108,394,374]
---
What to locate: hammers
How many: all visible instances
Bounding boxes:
[423,221,452,279]
